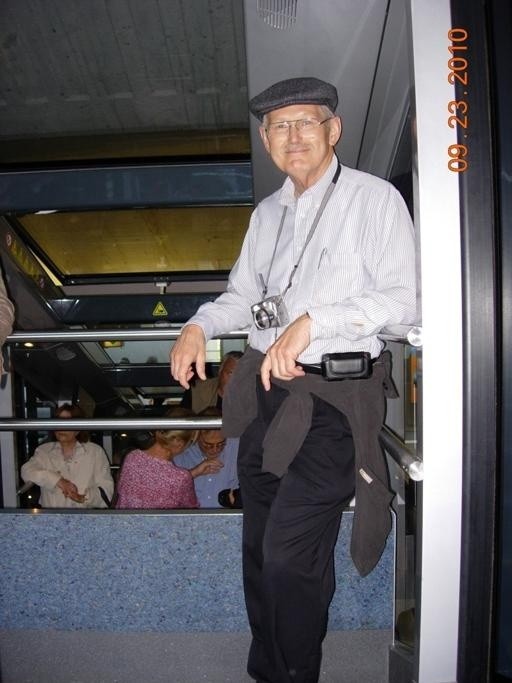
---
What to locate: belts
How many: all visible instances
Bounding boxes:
[295,357,378,374]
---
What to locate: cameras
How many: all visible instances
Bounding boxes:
[250,295,290,331]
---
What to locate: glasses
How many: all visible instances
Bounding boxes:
[262,117,333,135]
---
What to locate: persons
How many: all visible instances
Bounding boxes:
[179,348,245,415]
[18,403,114,512]
[163,69,424,682]
[170,405,243,509]
[109,406,201,512]
[0,264,18,377]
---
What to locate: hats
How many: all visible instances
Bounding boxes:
[247,77,339,122]
